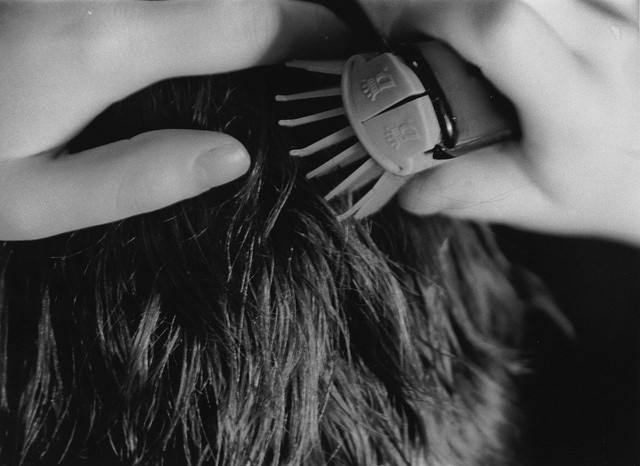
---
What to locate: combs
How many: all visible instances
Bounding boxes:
[276,37,517,221]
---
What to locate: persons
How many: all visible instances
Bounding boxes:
[0,0,639,466]
